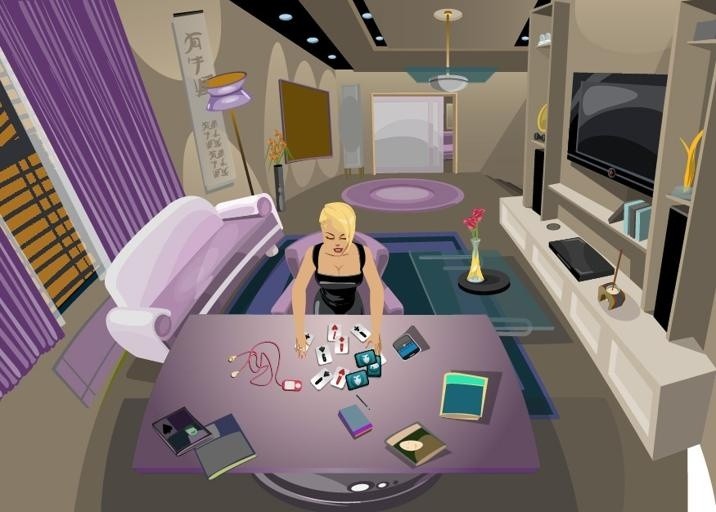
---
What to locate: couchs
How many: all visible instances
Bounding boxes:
[105,192,286,363]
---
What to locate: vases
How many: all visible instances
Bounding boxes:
[274,166,286,210]
[466,240,486,283]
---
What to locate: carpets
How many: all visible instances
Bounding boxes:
[341,178,463,213]
[224,231,560,419]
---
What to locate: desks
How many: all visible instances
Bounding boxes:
[134,313,540,512]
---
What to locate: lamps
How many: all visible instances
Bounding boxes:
[203,72,255,195]
[429,8,468,92]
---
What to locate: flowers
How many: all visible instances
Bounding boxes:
[265,130,292,166]
[464,208,486,239]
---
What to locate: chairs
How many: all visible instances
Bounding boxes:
[271,230,403,316]
